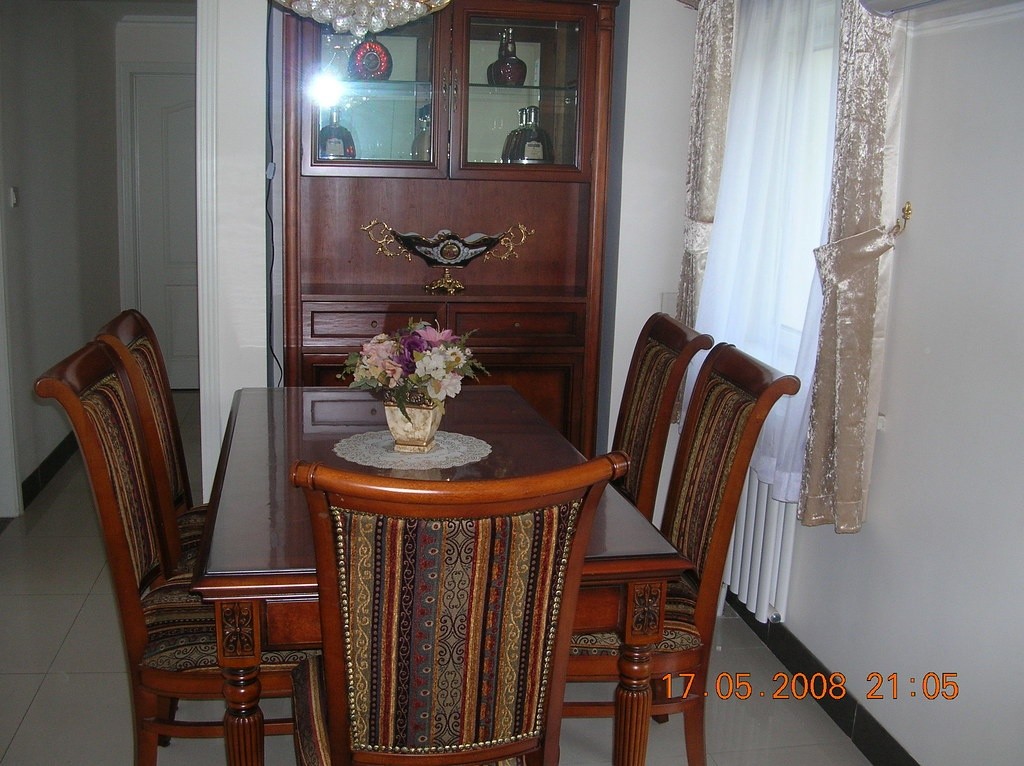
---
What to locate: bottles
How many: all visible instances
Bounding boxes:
[500,105,555,165]
[348,31,393,81]
[411,103,431,160]
[486,28,527,87]
[319,105,357,160]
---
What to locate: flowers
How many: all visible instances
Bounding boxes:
[336,317,493,425]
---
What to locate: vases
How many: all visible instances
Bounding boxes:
[380,387,445,454]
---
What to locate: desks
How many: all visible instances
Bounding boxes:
[188,385,697,766]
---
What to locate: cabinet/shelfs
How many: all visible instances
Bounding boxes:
[265,0,621,460]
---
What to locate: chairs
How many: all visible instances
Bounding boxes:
[34,307,321,766]
[554,311,801,766]
[290,449,631,766]
